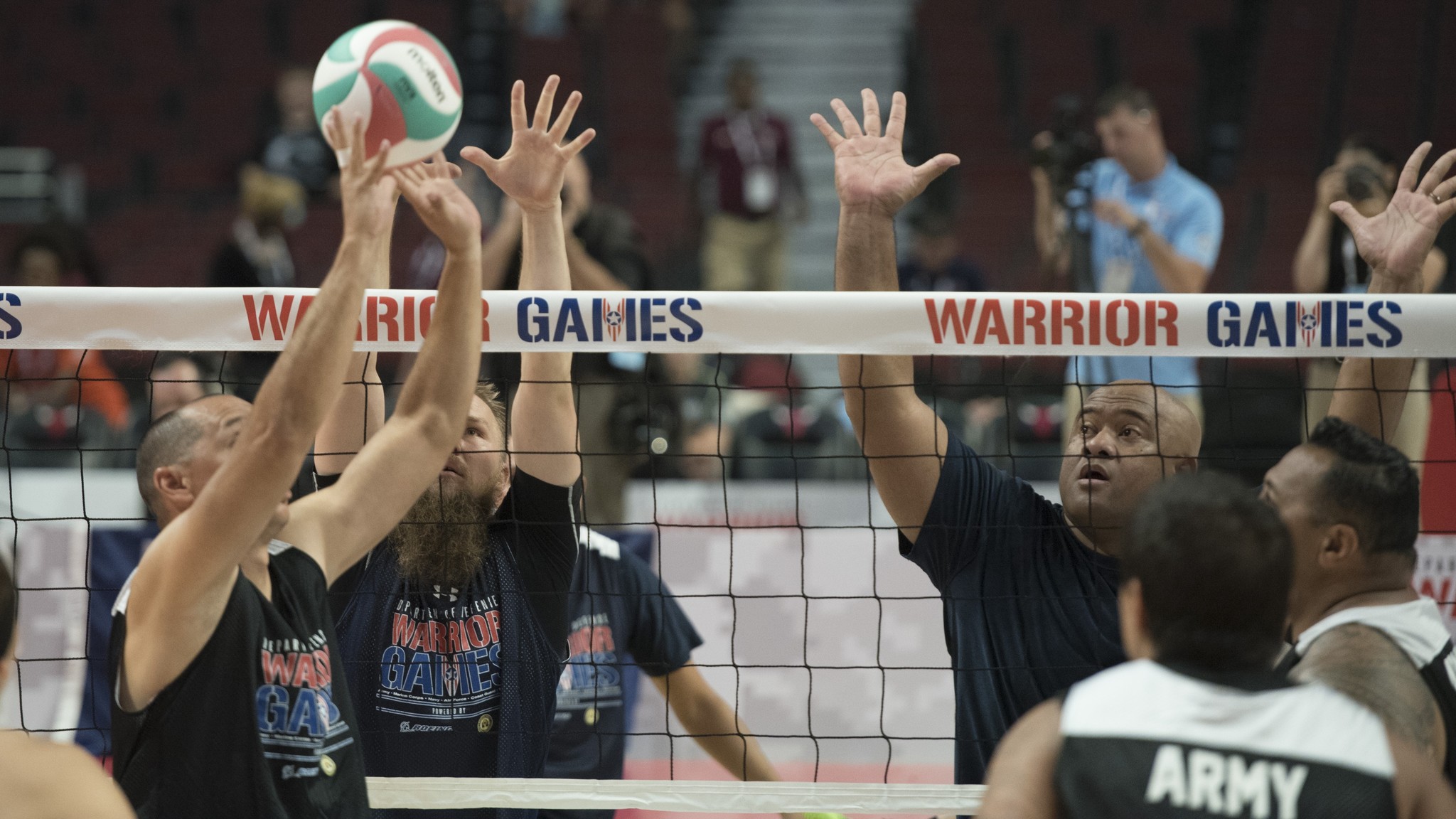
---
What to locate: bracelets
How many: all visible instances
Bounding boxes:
[1128,219,1146,240]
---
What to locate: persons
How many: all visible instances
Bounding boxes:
[0,53,809,819]
[808,69,1456,819]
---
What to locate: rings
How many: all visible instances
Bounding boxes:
[335,147,352,169]
[1428,191,1440,204]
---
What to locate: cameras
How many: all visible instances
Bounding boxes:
[1334,160,1384,199]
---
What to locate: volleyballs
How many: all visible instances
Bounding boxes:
[310,19,465,172]
